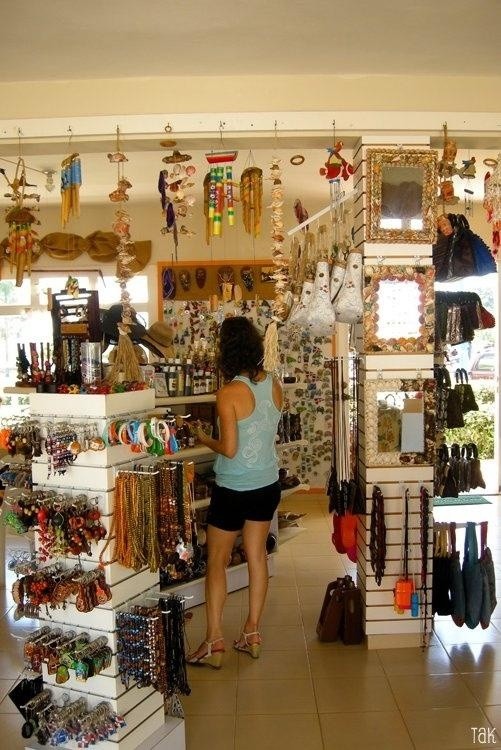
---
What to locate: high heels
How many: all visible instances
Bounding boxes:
[232,630,262,658]
[186,635,225,668]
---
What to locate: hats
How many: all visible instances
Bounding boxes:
[99,304,145,342]
[139,321,176,362]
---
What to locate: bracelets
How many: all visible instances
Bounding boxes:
[104,415,180,459]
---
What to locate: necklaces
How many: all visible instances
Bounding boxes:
[98,459,196,701]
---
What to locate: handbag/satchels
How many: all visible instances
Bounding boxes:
[432,522,451,616]
[442,369,464,429]
[325,465,366,515]
[434,443,458,498]
[451,522,465,626]
[462,521,483,628]
[459,444,471,492]
[450,443,465,492]
[475,293,494,328]
[461,214,496,276]
[456,367,479,413]
[435,291,474,345]
[432,213,458,280]
[446,213,473,281]
[481,522,497,628]
[434,368,449,429]
[341,576,363,643]
[468,442,486,489]
[315,574,342,640]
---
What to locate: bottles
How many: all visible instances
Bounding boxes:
[158,356,218,396]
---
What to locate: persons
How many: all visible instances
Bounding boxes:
[183,313,292,672]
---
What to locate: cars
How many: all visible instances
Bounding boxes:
[467,347,495,380]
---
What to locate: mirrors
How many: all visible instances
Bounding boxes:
[365,148,437,245]
[363,265,434,356]
[363,380,436,470]
